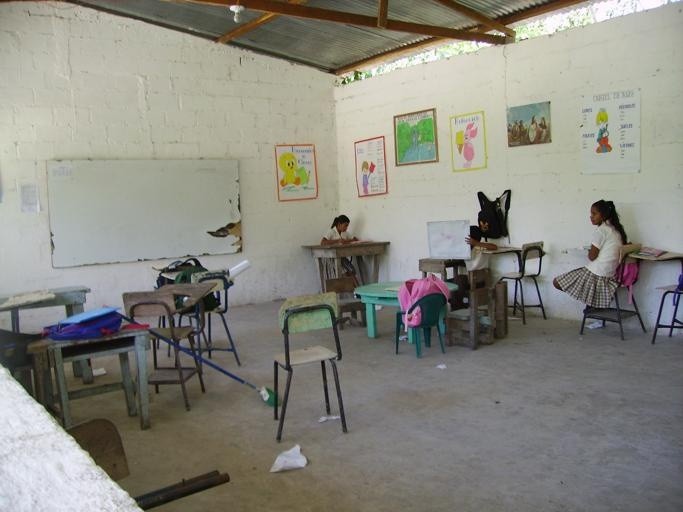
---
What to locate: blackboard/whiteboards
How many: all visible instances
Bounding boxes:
[428,220,472,260]
[46,157,243,268]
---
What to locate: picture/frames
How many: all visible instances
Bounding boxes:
[394,108,438,166]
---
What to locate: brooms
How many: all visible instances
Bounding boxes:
[102,304,282,409]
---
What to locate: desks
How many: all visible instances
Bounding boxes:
[2,287,97,385]
[355,279,459,338]
[630,244,683,272]
[483,247,522,270]
[445,270,496,350]
[419,257,467,283]
[45,323,152,431]
[305,239,390,293]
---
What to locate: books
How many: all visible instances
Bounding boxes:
[638,247,664,257]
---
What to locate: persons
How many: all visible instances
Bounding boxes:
[449,226,497,308]
[513,116,548,144]
[553,199,626,329]
[321,213,383,288]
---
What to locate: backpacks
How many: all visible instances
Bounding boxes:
[149,258,232,314]
[616,262,639,305]
[397,273,448,328]
[470,188,514,237]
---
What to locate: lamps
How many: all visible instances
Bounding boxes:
[229,5,245,23]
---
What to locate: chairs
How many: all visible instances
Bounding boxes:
[576,246,647,341]
[270,293,347,441]
[494,241,547,324]
[0,329,53,405]
[324,275,368,330]
[122,265,245,410]
[648,272,683,346]
[395,293,448,358]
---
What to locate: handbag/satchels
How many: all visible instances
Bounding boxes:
[42,307,129,339]
